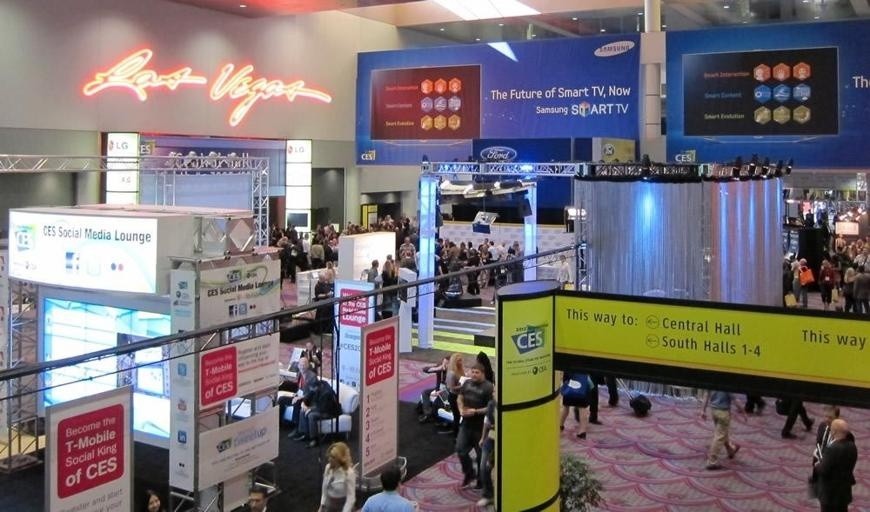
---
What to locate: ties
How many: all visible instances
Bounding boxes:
[821,426,831,452]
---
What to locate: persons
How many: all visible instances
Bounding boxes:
[701,389,749,469]
[805,419,858,511]
[316,441,357,511]
[811,405,858,484]
[736,392,768,415]
[556,254,572,290]
[269,213,524,331]
[559,369,595,441]
[605,374,619,408]
[574,372,602,425]
[781,204,870,315]
[775,397,815,439]
[292,373,342,448]
[238,484,278,511]
[419,350,497,507]
[279,357,317,436]
[360,466,418,511]
[301,339,321,374]
[137,489,165,511]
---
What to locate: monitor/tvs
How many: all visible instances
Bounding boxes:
[287,213,308,227]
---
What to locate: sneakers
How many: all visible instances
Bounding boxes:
[559,394,620,439]
[705,442,741,471]
[779,416,816,439]
[418,409,455,436]
[737,399,769,416]
[458,468,495,508]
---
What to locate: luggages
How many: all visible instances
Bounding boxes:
[614,377,652,418]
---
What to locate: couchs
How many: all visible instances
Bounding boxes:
[226,369,359,442]
[421,376,487,423]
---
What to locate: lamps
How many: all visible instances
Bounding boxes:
[166,151,237,167]
[640,154,650,180]
[500,180,521,189]
[732,156,793,180]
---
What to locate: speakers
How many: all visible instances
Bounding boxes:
[517,197,533,219]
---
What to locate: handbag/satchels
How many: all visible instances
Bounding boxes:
[798,265,816,287]
[807,467,820,500]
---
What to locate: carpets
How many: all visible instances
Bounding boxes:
[0,400,457,512]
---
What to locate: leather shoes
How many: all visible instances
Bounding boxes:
[286,427,321,450]
